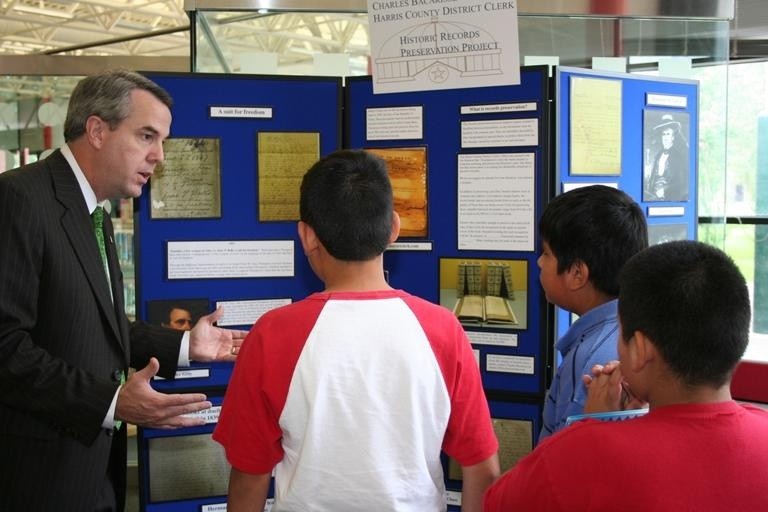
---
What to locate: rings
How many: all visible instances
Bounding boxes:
[230,346,236,355]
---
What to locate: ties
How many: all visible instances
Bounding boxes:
[92,206,125,430]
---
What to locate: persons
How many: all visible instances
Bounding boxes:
[533,181,650,442]
[207,147,503,510]
[478,239,768,512]
[1,67,252,512]
[640,109,691,202]
[156,302,192,333]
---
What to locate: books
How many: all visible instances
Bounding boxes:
[448,260,518,326]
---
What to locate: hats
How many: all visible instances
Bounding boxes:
[653,114,680,132]
